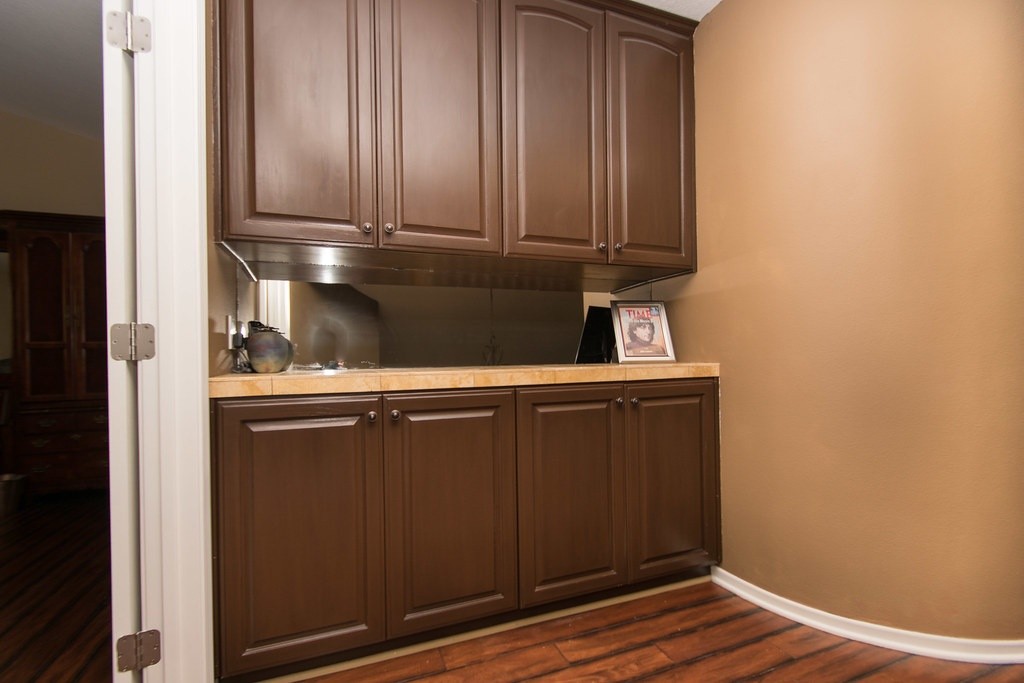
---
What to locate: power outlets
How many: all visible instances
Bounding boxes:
[227,314,237,350]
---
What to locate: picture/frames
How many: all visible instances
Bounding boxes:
[610,299,676,363]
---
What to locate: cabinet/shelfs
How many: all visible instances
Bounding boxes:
[12,231,109,504]
[223,0,698,283]
[210,378,722,683]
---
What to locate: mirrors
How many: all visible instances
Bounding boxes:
[234,260,652,369]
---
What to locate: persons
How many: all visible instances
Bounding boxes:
[626,316,665,354]
